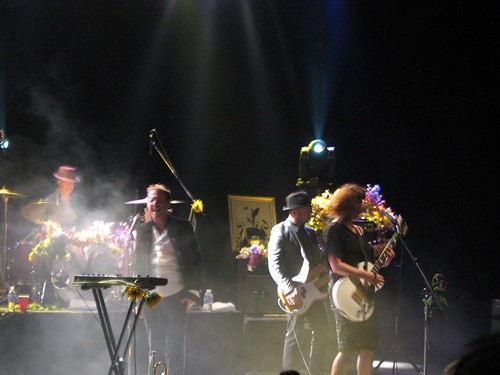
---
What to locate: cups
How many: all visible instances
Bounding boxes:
[18,295,30,311]
[250,236,259,246]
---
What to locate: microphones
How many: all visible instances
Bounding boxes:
[381,209,397,224]
[148,130,153,156]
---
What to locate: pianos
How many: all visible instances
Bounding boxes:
[73,270,170,375]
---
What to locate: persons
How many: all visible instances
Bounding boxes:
[267,192,329,375]
[135,185,201,375]
[44,166,87,224]
[325,184,396,375]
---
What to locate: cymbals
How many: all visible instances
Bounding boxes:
[124,199,186,206]
[19,199,72,229]
[0,188,25,200]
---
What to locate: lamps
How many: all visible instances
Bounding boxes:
[300,141,335,163]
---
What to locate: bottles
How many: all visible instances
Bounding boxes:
[203,289,213,311]
[8,286,16,311]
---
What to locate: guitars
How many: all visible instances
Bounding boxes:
[277,263,332,317]
[328,227,402,322]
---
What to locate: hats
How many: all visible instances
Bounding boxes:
[53,166,80,183]
[282,191,310,211]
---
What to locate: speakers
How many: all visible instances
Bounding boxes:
[236,262,286,316]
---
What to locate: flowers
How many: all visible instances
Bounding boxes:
[237,238,267,270]
[191,200,204,213]
[124,280,161,309]
[307,184,398,241]
[13,220,137,269]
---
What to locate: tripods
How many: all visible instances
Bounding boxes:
[370,226,441,374]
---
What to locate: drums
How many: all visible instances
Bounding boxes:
[50,240,123,306]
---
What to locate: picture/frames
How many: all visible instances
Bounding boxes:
[228,194,277,251]
